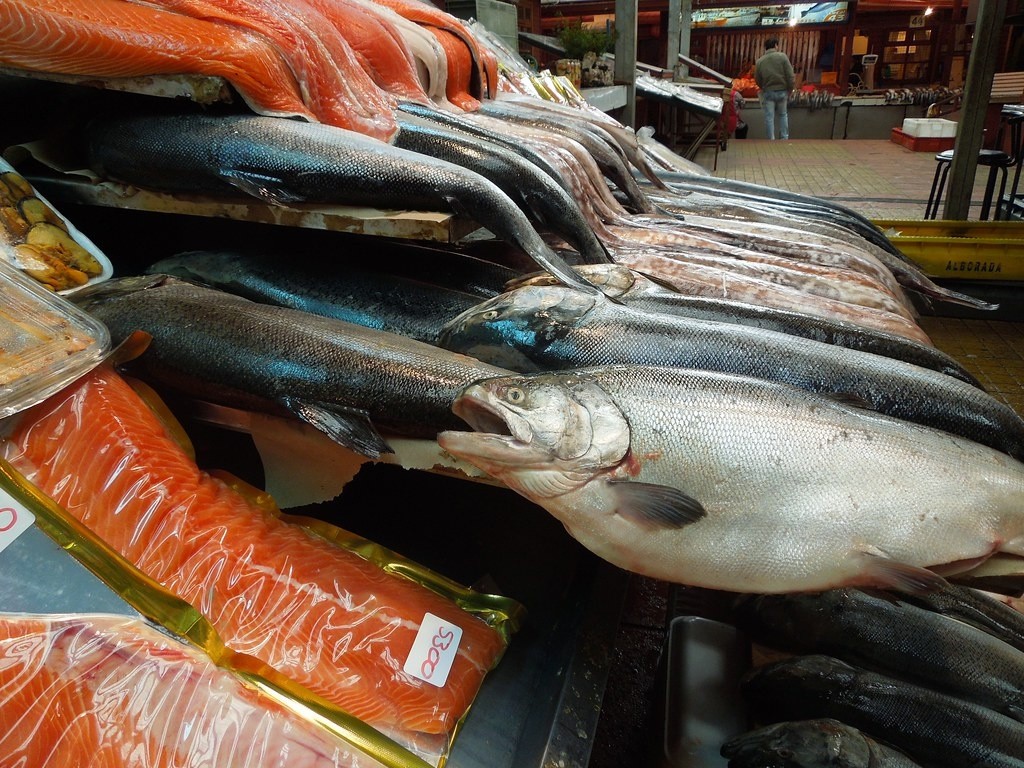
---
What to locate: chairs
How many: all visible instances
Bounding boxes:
[923,110,1024,220]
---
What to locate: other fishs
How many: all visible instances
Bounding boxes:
[1,0,1024,768]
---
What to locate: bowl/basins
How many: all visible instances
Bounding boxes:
[0,258,111,422]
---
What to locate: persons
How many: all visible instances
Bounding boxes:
[721,79,748,139]
[849,55,864,91]
[754,38,795,140]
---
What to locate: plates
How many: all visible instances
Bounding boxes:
[0,158,114,298]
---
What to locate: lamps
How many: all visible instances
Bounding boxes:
[862,54,879,65]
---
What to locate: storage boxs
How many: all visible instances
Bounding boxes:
[902,118,958,137]
[820,72,838,84]
